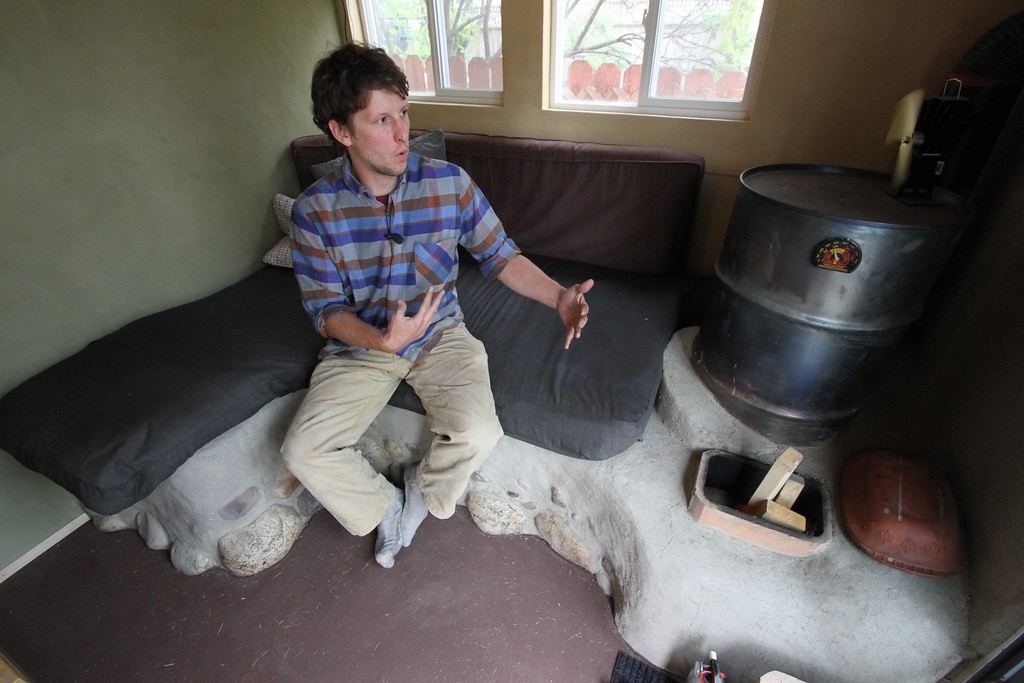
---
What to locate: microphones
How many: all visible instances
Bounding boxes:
[387,230,404,244]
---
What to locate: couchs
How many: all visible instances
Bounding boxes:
[1,130,705,516]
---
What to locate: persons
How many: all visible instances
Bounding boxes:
[278,40,598,571]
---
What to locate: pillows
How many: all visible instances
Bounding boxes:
[263,193,296,269]
[308,129,447,182]
